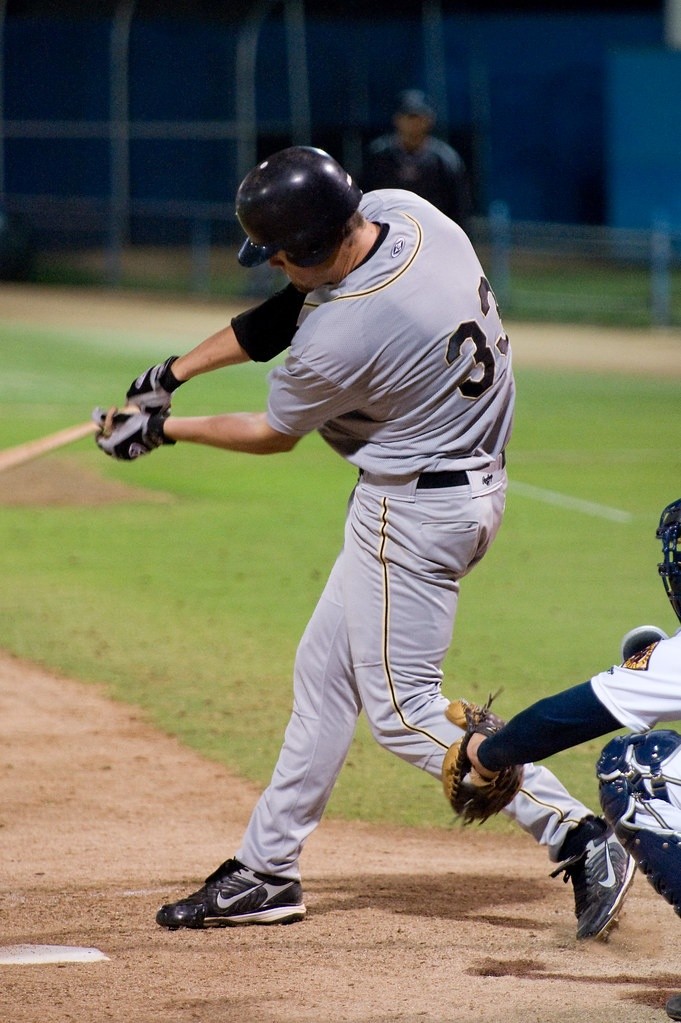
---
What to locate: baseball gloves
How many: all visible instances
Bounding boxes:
[441,697,526,821]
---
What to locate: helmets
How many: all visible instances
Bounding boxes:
[237,146,362,268]
[656,497,681,624]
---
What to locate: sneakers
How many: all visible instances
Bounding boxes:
[549,815,637,945]
[156,859,306,928]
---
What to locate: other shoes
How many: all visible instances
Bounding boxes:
[666,996,681,1021]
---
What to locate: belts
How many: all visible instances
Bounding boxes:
[359,449,506,489]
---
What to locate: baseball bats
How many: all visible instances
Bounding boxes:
[0,402,142,474]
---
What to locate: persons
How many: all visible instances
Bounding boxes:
[463,499,680,1021]
[348,87,469,236]
[92,146,637,942]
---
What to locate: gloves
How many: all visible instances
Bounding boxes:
[92,406,176,460]
[126,356,187,415]
